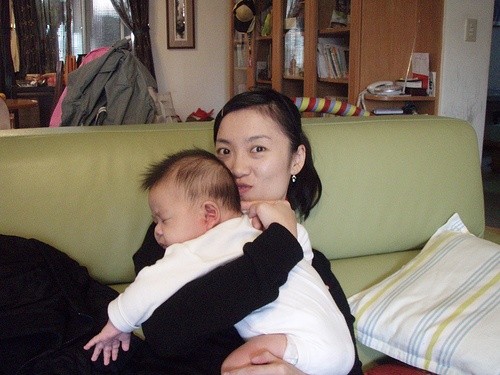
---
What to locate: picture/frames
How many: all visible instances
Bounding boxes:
[166,0,195,49]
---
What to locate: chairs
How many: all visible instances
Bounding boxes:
[1,48,35,97]
[48,55,78,120]
[0,93,14,129]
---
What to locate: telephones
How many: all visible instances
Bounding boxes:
[366,80,402,95]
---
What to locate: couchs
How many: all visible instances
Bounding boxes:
[0,115,500,370]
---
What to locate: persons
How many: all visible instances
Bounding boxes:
[83,86,364,375]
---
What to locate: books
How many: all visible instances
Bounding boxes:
[317,37,349,80]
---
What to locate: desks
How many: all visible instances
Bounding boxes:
[12,84,55,128]
[6,99,40,128]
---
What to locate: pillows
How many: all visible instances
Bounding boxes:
[346,212,500,375]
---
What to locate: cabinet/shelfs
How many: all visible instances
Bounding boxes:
[226,0,445,117]
